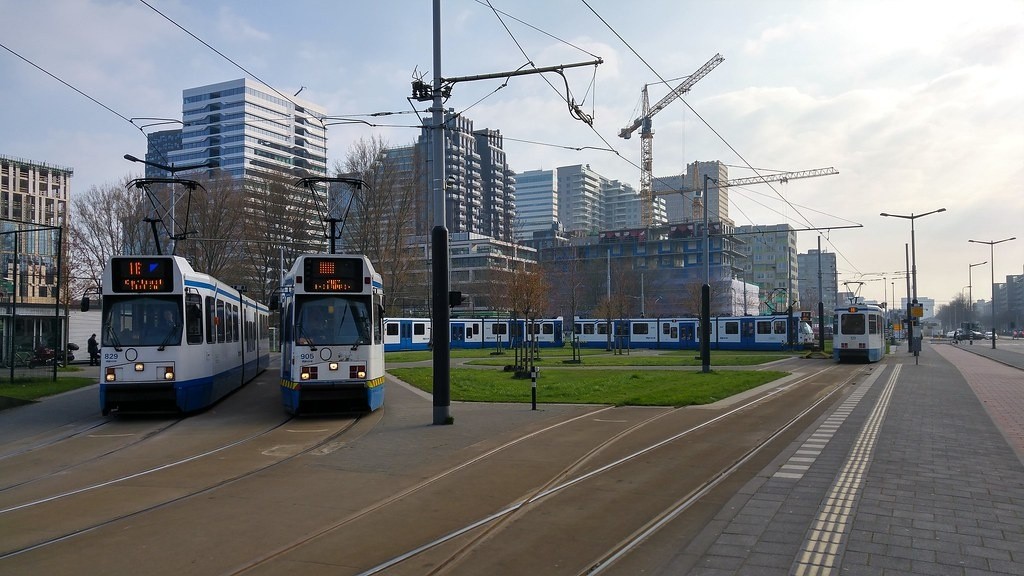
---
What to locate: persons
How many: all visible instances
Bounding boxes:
[954,331,958,345]
[88,334,99,366]
[160,310,180,328]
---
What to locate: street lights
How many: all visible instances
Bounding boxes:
[969,262,988,330]
[880,207,947,356]
[968,237,1017,349]
[883,277,894,314]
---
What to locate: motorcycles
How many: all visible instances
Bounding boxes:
[27,336,79,368]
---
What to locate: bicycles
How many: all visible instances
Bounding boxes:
[950,335,966,346]
[3,342,41,369]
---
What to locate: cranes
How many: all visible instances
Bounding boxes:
[617,52,726,228]
[635,160,841,222]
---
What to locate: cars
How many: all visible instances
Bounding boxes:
[946,328,998,341]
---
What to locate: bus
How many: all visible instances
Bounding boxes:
[829,304,887,363]
[80,254,270,419]
[268,253,815,418]
